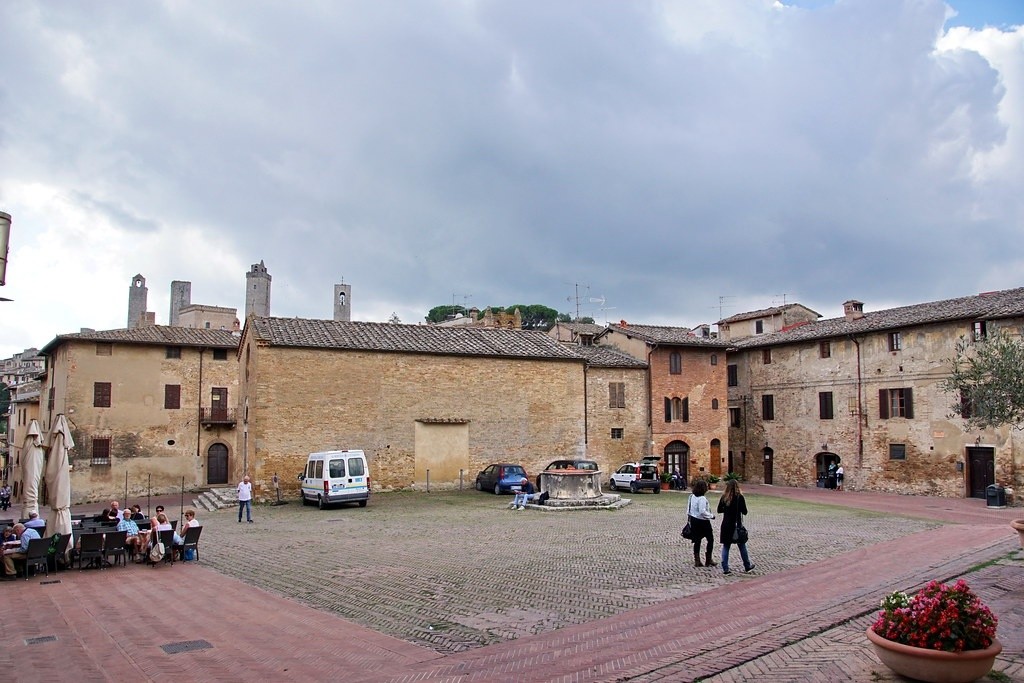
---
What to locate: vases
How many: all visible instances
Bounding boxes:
[866,627,1002,683]
[1011,519,1024,547]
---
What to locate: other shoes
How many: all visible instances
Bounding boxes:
[238,518,242,522]
[247,519,254,523]
[0,574,16,581]
[517,505,526,511]
[724,570,733,574]
[510,505,518,510]
[745,563,756,572]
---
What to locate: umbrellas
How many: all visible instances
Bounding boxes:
[42,413,76,570]
[21,418,44,519]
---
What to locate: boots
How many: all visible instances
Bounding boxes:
[693,552,704,567]
[704,552,718,566]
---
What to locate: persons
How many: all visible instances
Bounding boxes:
[1,480,11,511]
[237,476,252,523]
[717,479,755,576]
[828,461,843,491]
[673,467,686,490]
[94,500,200,562]
[511,478,536,511]
[686,481,718,567]
[0,510,46,580]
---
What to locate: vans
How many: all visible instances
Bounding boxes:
[536,459,597,494]
[298,449,370,508]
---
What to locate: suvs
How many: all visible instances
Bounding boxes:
[610,461,662,494]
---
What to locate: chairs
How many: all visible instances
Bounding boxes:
[0,514,203,580]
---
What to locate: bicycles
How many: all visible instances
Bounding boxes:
[1,496,10,511]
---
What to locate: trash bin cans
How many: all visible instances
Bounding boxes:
[986,484,1006,506]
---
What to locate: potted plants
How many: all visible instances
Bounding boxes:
[660,473,671,490]
[709,475,721,491]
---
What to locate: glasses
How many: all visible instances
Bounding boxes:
[156,510,163,513]
[185,514,189,517]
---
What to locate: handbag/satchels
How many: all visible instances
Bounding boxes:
[730,523,750,545]
[679,522,693,540]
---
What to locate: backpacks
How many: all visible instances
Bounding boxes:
[149,526,165,563]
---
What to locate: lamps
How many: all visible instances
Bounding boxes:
[848,396,868,427]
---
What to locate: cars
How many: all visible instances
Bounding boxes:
[0,496,11,508]
[477,463,529,496]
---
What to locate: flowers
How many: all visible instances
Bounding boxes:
[871,579,999,654]
